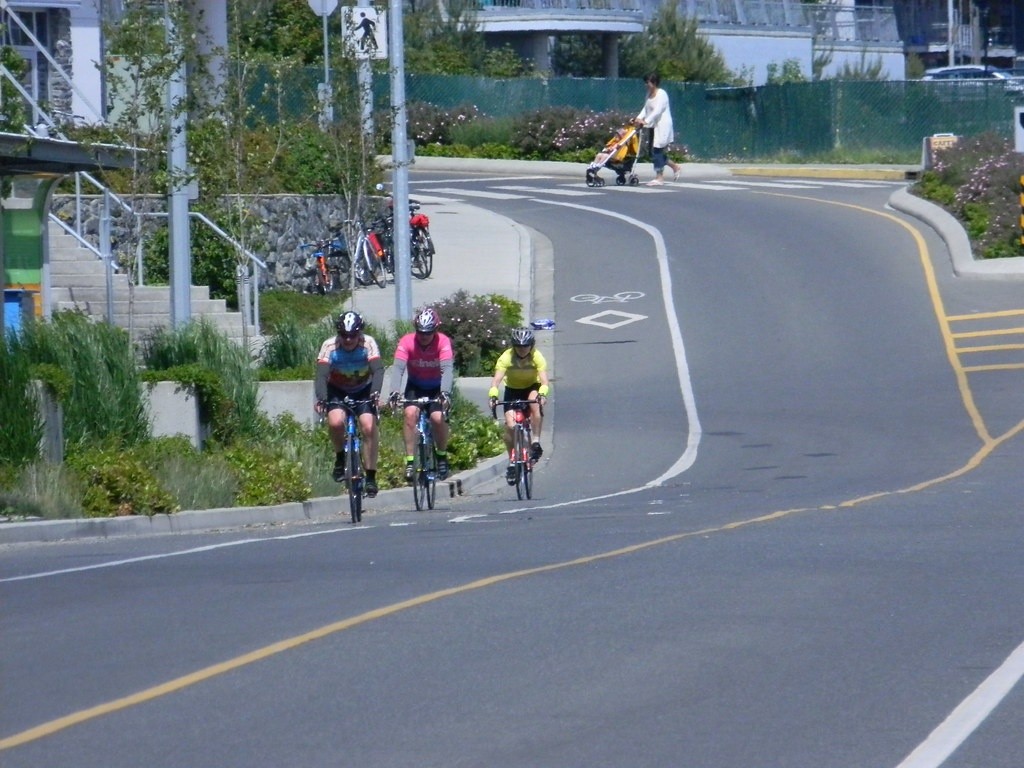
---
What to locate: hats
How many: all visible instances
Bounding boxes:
[616,130,628,139]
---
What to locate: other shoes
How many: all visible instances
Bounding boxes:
[646,180,665,186]
[674,164,683,182]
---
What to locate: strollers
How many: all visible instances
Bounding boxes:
[585,118,646,188]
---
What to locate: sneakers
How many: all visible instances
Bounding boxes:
[531,442,543,463]
[365,482,378,494]
[333,461,346,483]
[435,448,449,481]
[505,466,517,486]
[404,461,414,482]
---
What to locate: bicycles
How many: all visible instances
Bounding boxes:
[386,391,450,510]
[302,195,436,294]
[487,395,549,500]
[316,393,374,520]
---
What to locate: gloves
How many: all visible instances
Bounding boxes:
[389,392,400,410]
[440,391,451,412]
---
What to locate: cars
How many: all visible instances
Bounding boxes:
[922,64,1024,95]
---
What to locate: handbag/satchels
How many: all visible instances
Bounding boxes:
[410,213,430,229]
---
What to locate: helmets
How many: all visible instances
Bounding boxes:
[510,327,536,346]
[337,311,365,333]
[412,308,441,332]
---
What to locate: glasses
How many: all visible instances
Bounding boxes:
[415,330,434,336]
[511,343,531,348]
[337,330,359,339]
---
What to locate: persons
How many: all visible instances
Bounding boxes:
[629,75,680,186]
[390,309,453,480]
[589,131,626,168]
[489,326,548,485]
[314,312,385,496]
